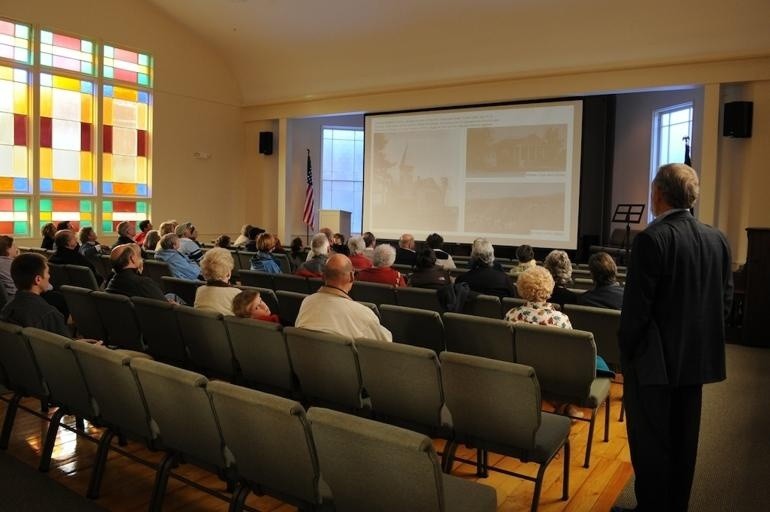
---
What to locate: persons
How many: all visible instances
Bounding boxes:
[610,164,734,512]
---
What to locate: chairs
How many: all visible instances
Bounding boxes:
[1,236,631,512]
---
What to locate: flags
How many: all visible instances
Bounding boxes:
[302,156,315,231]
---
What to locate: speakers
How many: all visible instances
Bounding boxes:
[259,131,273,155]
[722,101,754,138]
